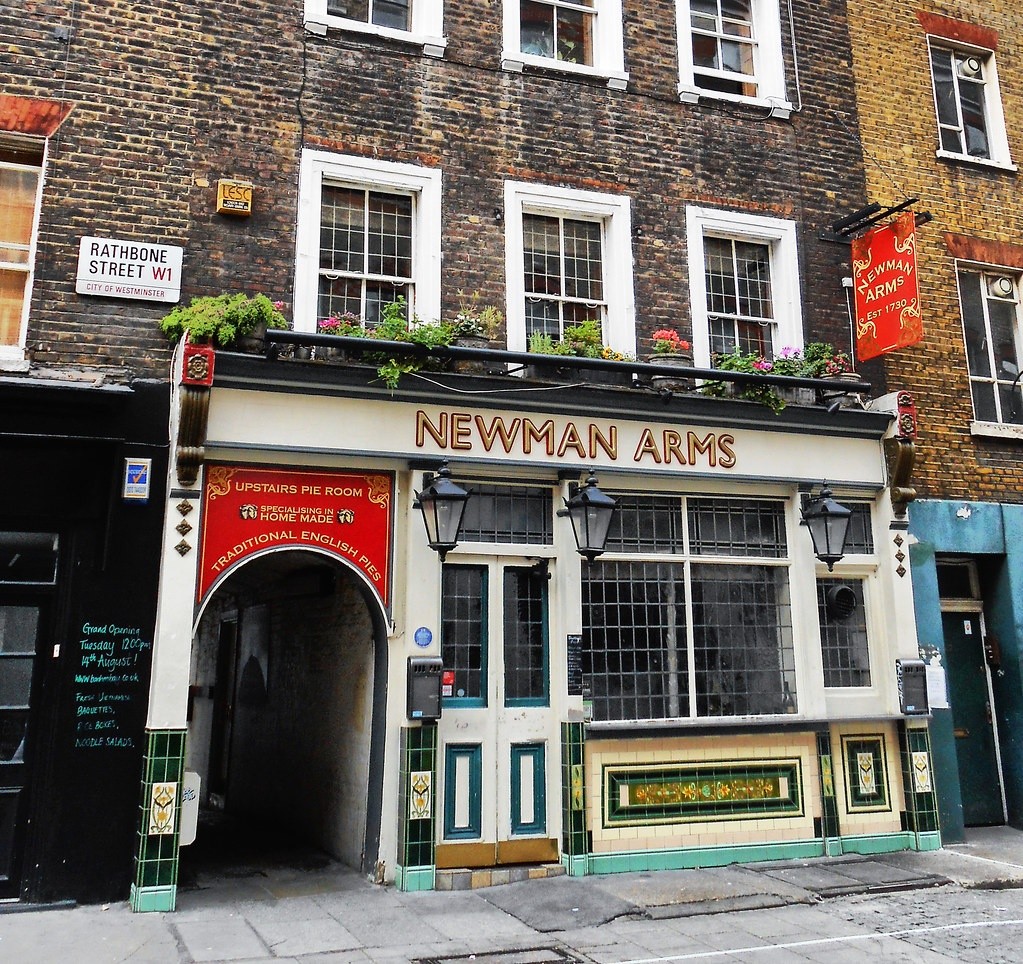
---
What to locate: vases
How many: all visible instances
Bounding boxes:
[722,372,752,400]
[227,315,268,355]
[647,352,692,391]
[450,335,492,377]
[817,371,863,408]
[774,377,816,407]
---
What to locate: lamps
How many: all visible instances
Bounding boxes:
[799,478,857,574]
[412,454,475,566]
[560,465,622,566]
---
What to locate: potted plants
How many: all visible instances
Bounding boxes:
[523,330,560,381]
[603,345,635,387]
[559,319,604,383]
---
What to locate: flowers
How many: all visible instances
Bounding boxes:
[648,329,692,354]
[447,287,505,340]
[315,311,367,342]
[804,341,853,377]
[769,346,817,379]
[703,344,787,417]
[165,292,293,344]
